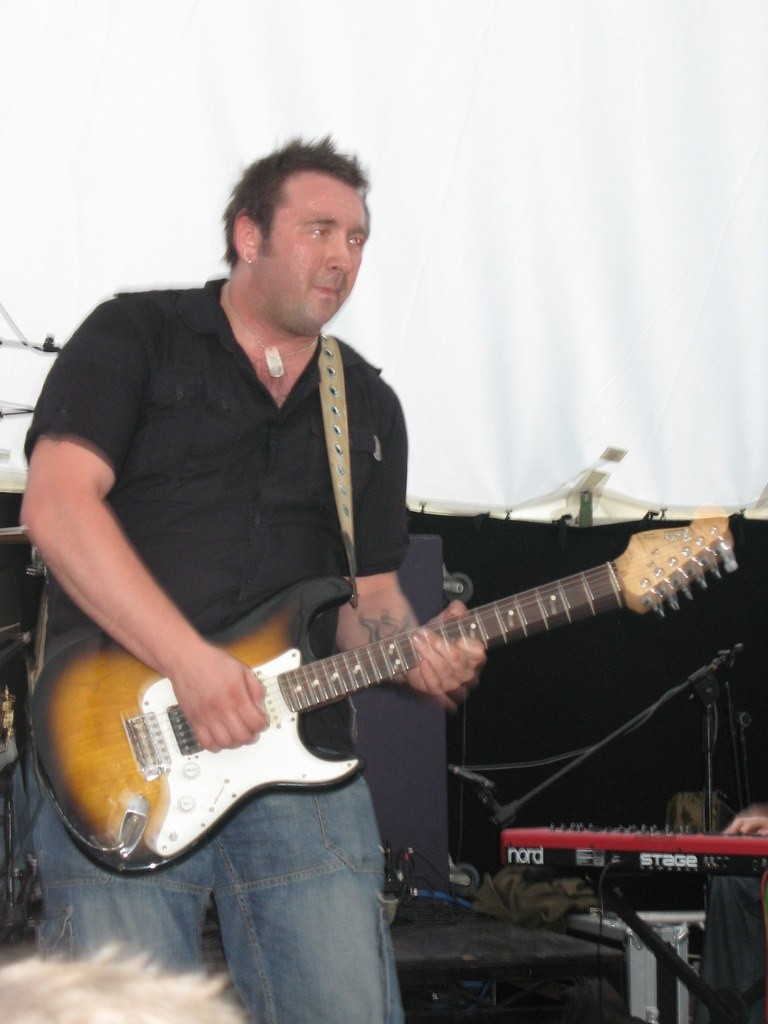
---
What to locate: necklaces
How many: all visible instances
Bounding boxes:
[226,281,318,377]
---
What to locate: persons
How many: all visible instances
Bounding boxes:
[699,806,768,1024]
[21,138,486,1023]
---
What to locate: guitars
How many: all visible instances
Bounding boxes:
[21,515,743,877]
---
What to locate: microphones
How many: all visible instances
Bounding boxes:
[448,765,495,791]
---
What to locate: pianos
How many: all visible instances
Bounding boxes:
[500,819,768,881]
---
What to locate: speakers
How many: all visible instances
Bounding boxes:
[347,536,453,918]
